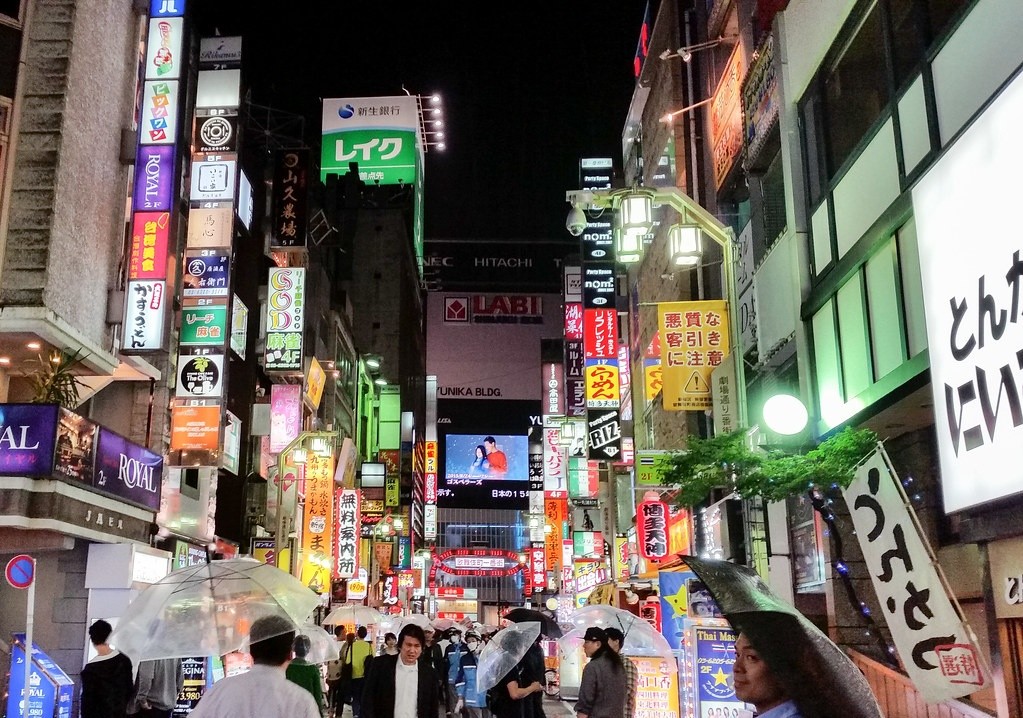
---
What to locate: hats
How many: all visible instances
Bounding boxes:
[576,627,608,642]
[449,629,463,635]
[424,625,435,632]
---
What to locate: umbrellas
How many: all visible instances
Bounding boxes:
[104,545,321,677]
[676,553,884,718]
[322,601,382,625]
[238,622,341,666]
[380,600,674,696]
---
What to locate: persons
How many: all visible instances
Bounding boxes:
[733,632,801,718]
[80,620,133,718]
[470,436,508,479]
[283,626,639,718]
[126,620,184,718]
[186,615,320,718]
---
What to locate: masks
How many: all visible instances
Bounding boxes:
[451,637,459,643]
[467,642,479,652]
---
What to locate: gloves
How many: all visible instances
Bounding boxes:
[455,700,463,714]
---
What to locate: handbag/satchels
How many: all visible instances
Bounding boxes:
[341,644,353,687]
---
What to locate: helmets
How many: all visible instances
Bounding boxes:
[465,629,481,640]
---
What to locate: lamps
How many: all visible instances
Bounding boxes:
[677,35,722,62]
[364,353,387,385]
[551,417,587,448]
[659,97,713,123]
[659,43,718,61]
[611,185,736,266]
[317,360,340,378]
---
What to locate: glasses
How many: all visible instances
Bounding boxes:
[424,631,433,635]
